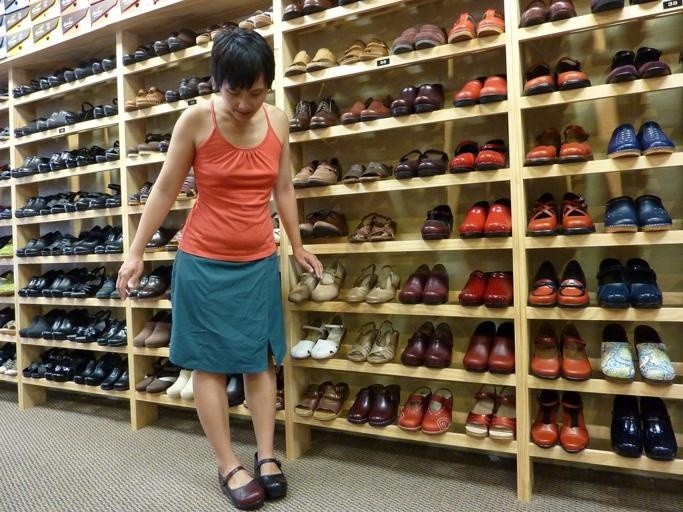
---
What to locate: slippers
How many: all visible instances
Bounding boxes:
[313,383,350,420]
[8,322,16,335]
[41,235,63,256]
[285,50,311,77]
[306,48,339,73]
[51,234,79,256]
[341,162,366,184]
[337,40,366,65]
[295,382,334,418]
[0,320,15,334]
[359,39,390,60]
[358,163,392,181]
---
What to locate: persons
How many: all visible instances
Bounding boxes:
[115,26,325,512]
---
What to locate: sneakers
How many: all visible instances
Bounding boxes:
[0,359,16,374]
[0,280,14,295]
[5,363,16,375]
[0,239,13,257]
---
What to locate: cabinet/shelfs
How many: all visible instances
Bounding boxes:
[0,59,20,412]
[277,0,524,504]
[116,0,288,461]
[8,19,134,435]
[510,1,683,503]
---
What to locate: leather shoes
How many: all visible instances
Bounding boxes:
[38,153,61,173]
[64,63,87,83]
[0,93,9,102]
[63,191,88,212]
[0,270,13,281]
[309,99,340,129]
[134,309,167,347]
[44,349,83,380]
[422,388,453,434]
[254,452,288,501]
[128,265,166,300]
[554,57,591,90]
[19,309,57,337]
[591,0,624,12]
[135,357,168,393]
[102,59,116,71]
[74,226,112,254]
[448,10,476,44]
[154,32,176,55]
[299,210,326,238]
[41,308,79,340]
[136,87,164,109]
[638,121,675,156]
[137,266,172,302]
[176,175,199,201]
[92,61,102,74]
[549,0,577,20]
[532,390,558,448]
[123,45,143,65]
[0,208,12,219]
[23,195,51,216]
[483,198,511,237]
[15,197,36,218]
[392,26,418,55]
[523,63,555,95]
[226,375,245,407]
[89,184,119,209]
[606,50,637,84]
[489,322,514,373]
[0,126,10,141]
[635,47,671,79]
[65,102,102,124]
[50,149,77,171]
[524,127,561,165]
[101,358,128,390]
[423,264,449,304]
[105,194,121,208]
[415,85,445,113]
[0,170,11,179]
[128,181,152,206]
[531,325,561,379]
[65,148,89,168]
[52,351,95,382]
[612,395,645,458]
[11,156,32,178]
[561,392,588,453]
[114,369,130,391]
[74,353,105,384]
[105,233,123,253]
[642,397,677,460]
[105,104,118,116]
[139,184,154,205]
[604,195,638,233]
[74,192,102,212]
[127,134,160,157]
[394,150,422,179]
[0,235,12,249]
[561,192,596,234]
[145,312,172,348]
[601,324,636,383]
[399,386,431,430]
[164,228,183,251]
[459,270,489,305]
[138,133,171,155]
[425,323,453,369]
[145,227,177,253]
[166,370,192,399]
[63,226,101,256]
[312,212,347,238]
[16,232,52,257]
[97,319,126,346]
[347,384,383,423]
[48,68,72,86]
[85,353,122,386]
[40,69,60,90]
[96,273,118,299]
[302,1,339,14]
[12,81,38,98]
[0,342,15,367]
[480,74,507,103]
[180,370,194,400]
[218,467,264,510]
[558,259,590,308]
[288,271,319,303]
[14,119,38,137]
[520,0,549,27]
[475,140,507,170]
[102,54,117,70]
[477,8,504,36]
[391,86,420,116]
[401,322,435,366]
[634,325,675,382]
[179,77,200,98]
[76,145,105,166]
[625,258,663,308]
[32,349,69,378]
[19,77,47,95]
[596,259,629,309]
[283,0,303,20]
[289,99,317,132]
[169,28,199,52]
[399,263,430,304]
[165,79,188,102]
[94,226,122,253]
[74,58,99,79]
[0,307,15,329]
[18,269,55,297]
[528,193,560,235]
[125,88,148,111]
[108,324,128,346]
[418,150,448,175]
[463,320,496,372]
[22,118,47,135]
[75,309,111,343]
[110,290,120,299]
[25,231,63,256]
[52,309,89,340]
[483,272,512,308]
[46,110,74,130]
[18,156,50,175]
[42,268,77,297]
[421,205,452,239]
[159,140,169,152]
[449,142,477,172]
[198,78,213,94]
[340,96,374,124]
[561,324,592,382]
[147,366,181,397]
[25,270,64,298]
[27,309,65,338]
[23,347,58,377]
[292,160,320,188]
[528,260,557,305]
[608,123,641,158]
[368,384,400,426]
[634,194,673,232]
[270,213,280,248]
[454,77,488,107]
[360,95,394,121]
[275,386,284,410]
[93,54,115,75]
[414,22,447,50]
[135,41,155,62]
[94,97,118,118]
[67,310,105,341]
[312,258,346,303]
[37,111,58,131]
[559,125,592,161]
[51,267,88,297]
[308,158,342,186]
[460,200,490,238]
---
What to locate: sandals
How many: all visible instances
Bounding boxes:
[489,387,517,440]
[367,265,400,303]
[466,385,503,438]
[291,316,328,359]
[62,266,99,297]
[367,320,400,364]
[40,192,67,215]
[196,25,218,45]
[96,140,119,163]
[312,314,347,360]
[240,10,263,28]
[347,211,377,242]
[369,213,398,241]
[51,192,76,213]
[255,6,272,28]
[345,264,378,302]
[347,321,379,361]
[71,266,106,298]
[211,21,238,39]
[106,147,119,160]
